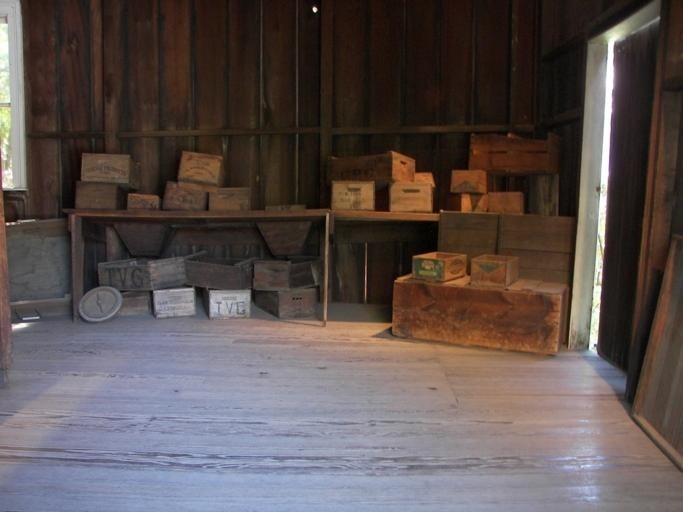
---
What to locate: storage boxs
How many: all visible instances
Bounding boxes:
[76,131,570,355]
